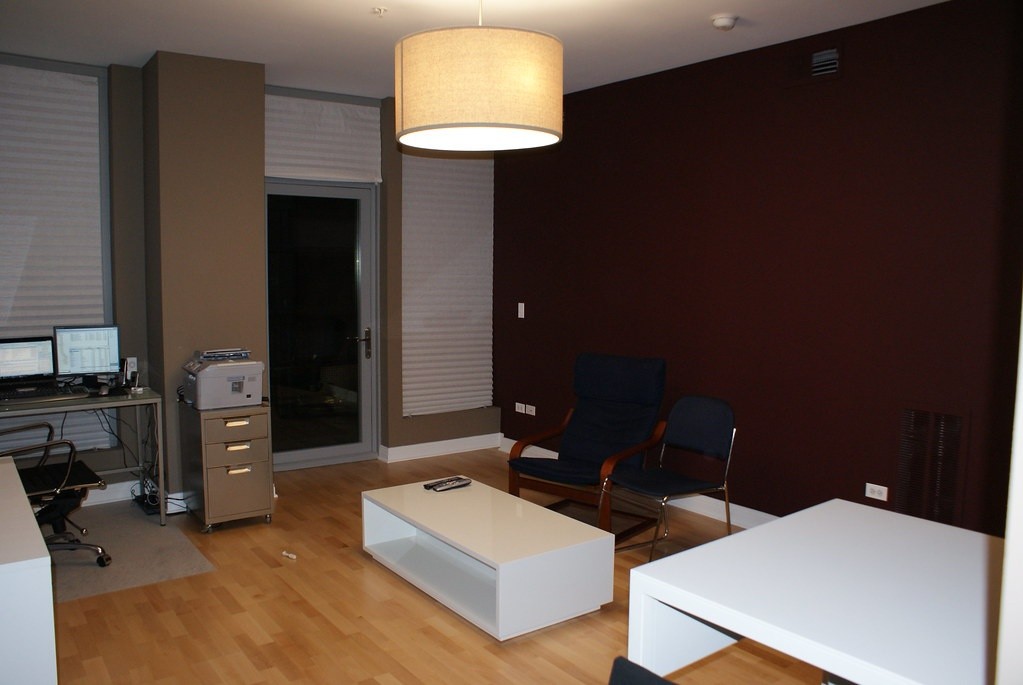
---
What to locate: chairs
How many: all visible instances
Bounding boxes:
[507,351,670,546]
[0,422,111,565]
[594,394,737,561]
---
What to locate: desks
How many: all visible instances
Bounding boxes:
[0,385,167,527]
[627,498,1005,685]
[361,474,615,642]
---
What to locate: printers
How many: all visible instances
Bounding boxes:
[177,347,265,410]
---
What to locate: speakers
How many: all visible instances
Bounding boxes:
[121,356,138,381]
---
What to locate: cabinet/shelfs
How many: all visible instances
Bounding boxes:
[179,399,273,531]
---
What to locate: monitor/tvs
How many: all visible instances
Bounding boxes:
[53,324,120,390]
[0,336,55,392]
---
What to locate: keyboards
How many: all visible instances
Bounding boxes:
[0,382,89,406]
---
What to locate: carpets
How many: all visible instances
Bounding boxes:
[38,497,215,601]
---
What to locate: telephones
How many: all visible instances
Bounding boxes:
[119,357,129,388]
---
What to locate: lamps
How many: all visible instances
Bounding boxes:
[394,0,565,158]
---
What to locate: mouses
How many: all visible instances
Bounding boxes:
[99,385,113,396]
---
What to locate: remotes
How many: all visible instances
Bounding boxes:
[424,476,472,491]
